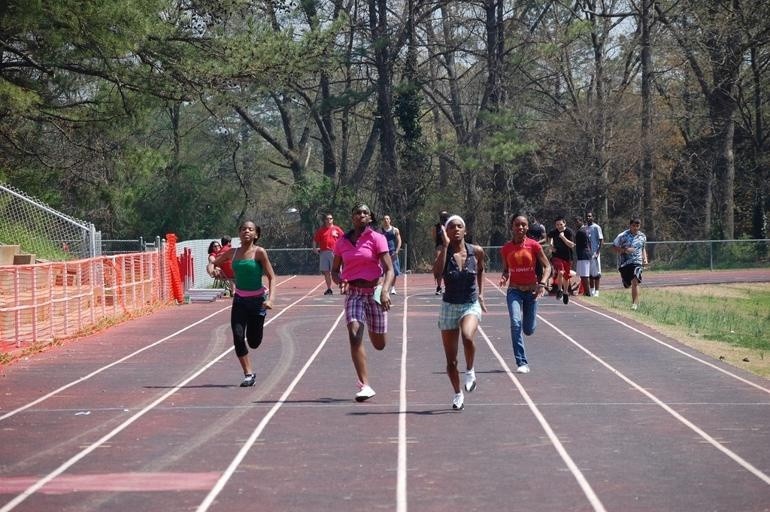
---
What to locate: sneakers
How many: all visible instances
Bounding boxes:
[557,289,563,299]
[240,372,256,387]
[436,285,442,295]
[324,289,332,295]
[516,365,530,374]
[563,294,568,304]
[452,390,465,410]
[630,303,638,311]
[390,286,396,294]
[354,380,376,403]
[464,367,477,393]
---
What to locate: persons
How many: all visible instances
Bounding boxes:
[216,235,236,297]
[214,219,275,387]
[313,213,348,295]
[499,213,551,373]
[525,210,549,295]
[547,217,576,304]
[586,211,603,297]
[611,219,648,310]
[433,215,488,410]
[332,203,395,401]
[574,216,591,296]
[208,241,221,264]
[432,210,450,295]
[377,215,402,294]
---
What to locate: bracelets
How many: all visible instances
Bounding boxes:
[624,247,628,254]
[538,281,545,287]
